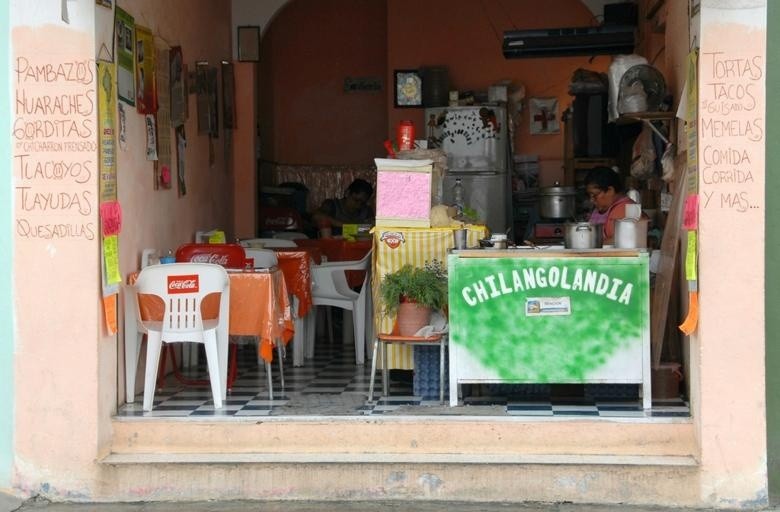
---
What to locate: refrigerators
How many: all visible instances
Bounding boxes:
[424,105,513,243]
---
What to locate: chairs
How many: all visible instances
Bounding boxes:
[121,239,448,413]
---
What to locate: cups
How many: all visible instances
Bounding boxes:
[251,243,264,248]
[158,256,176,265]
[317,225,332,239]
[452,228,467,249]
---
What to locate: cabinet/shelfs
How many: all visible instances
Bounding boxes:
[369,225,485,402]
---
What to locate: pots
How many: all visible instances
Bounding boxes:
[562,221,605,250]
[541,180,578,220]
[478,234,508,249]
[613,217,648,249]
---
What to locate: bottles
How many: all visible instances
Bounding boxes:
[452,177,465,207]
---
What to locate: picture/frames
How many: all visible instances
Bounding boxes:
[392,67,425,109]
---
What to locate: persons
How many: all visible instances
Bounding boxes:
[583,165,651,249]
[309,178,377,237]
